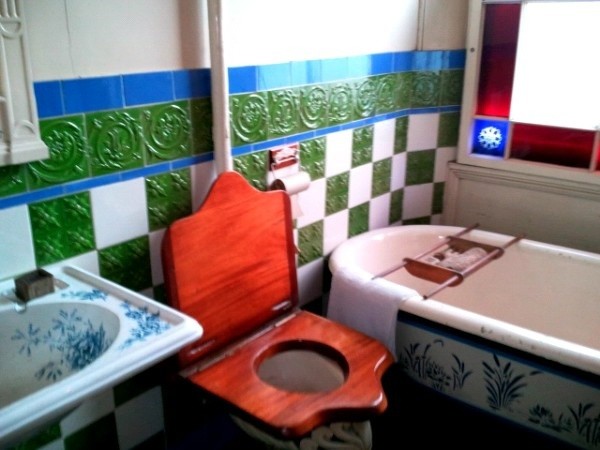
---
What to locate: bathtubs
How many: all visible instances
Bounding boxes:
[329,224,600,450]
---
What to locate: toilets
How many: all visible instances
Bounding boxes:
[161,170,396,450]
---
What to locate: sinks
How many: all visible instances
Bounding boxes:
[0,262,204,438]
